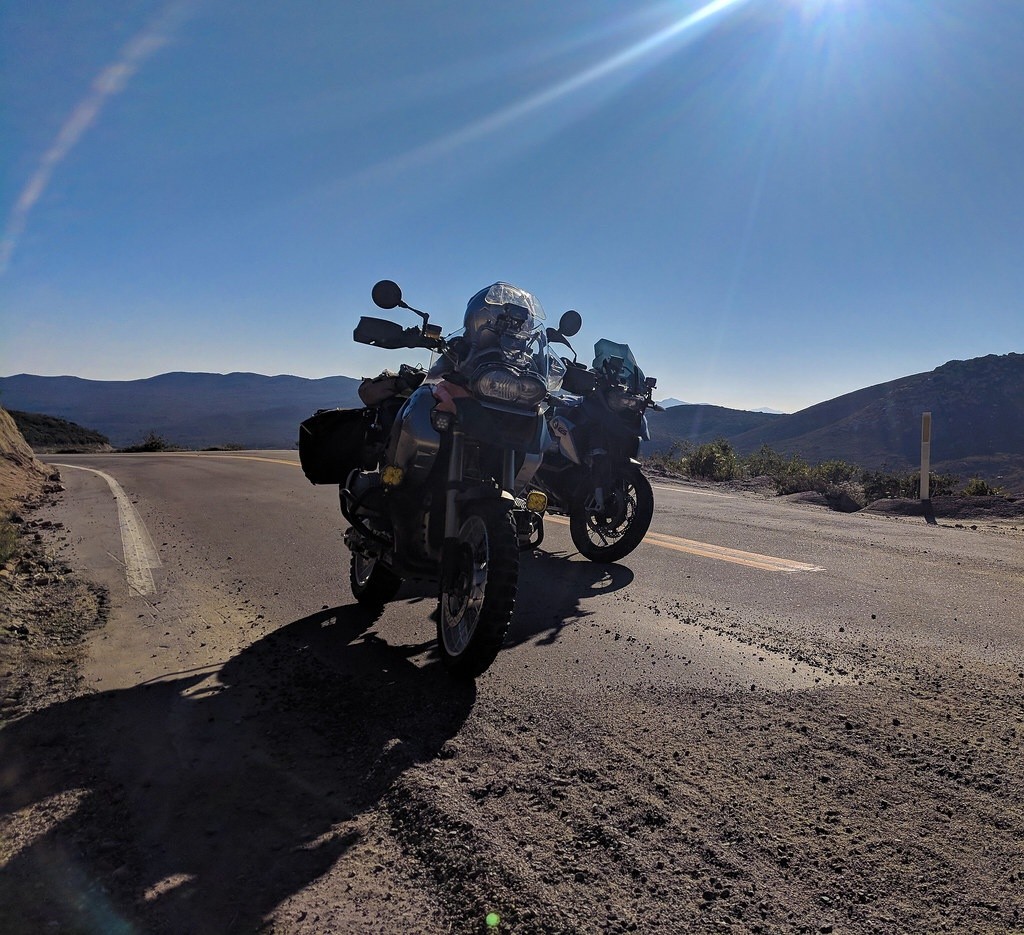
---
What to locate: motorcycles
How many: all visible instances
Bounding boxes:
[531,339,657,566]
[295,280,583,678]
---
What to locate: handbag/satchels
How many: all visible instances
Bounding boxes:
[300,400,374,488]
